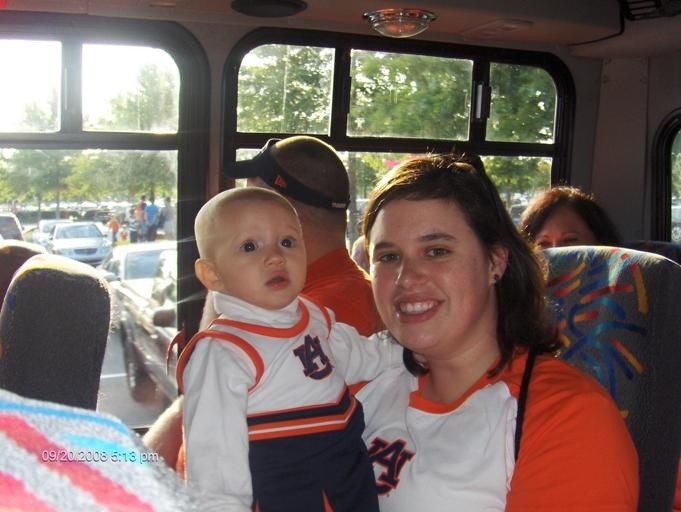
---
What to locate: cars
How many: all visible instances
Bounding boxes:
[500,191,532,228]
[340,197,374,239]
[1,199,182,406]
[667,198,681,251]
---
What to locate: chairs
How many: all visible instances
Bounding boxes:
[541,239,679,512]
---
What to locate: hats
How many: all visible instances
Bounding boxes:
[220,138,351,212]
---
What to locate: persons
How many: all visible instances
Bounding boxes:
[138,136,387,480]
[353,155,644,511]
[516,185,622,253]
[107,194,176,244]
[174,185,432,511]
